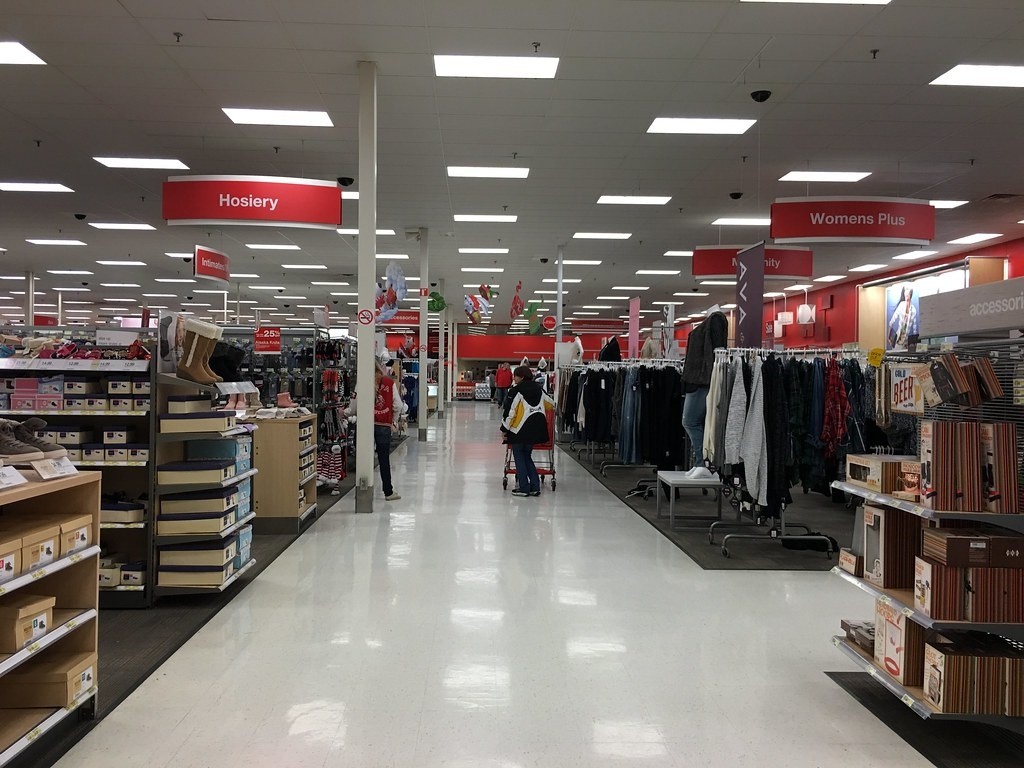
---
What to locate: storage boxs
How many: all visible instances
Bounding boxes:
[97,500,144,524]
[158,394,237,433]
[1,512,93,584]
[0,374,150,411]
[299,486,307,509]
[299,451,315,480]
[99,553,146,589]
[0,595,56,653]
[34,428,148,464]
[299,421,313,449]
[3,652,98,708]
[156,435,252,587]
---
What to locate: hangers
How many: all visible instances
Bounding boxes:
[554,358,684,376]
[713,347,862,370]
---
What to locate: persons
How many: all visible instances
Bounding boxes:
[486,373,490,383]
[171,314,186,372]
[499,365,555,497]
[490,370,496,404]
[344,355,403,501]
[888,286,917,350]
[494,363,513,409]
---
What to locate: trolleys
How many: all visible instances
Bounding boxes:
[502,407,557,492]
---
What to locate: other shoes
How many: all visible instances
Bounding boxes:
[530,490,541,496]
[385,492,402,501]
[511,489,529,497]
[498,405,501,409]
[21,336,152,360]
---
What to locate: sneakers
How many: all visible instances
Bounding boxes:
[0,419,45,461]
[9,419,67,457]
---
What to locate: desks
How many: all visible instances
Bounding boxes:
[655,472,742,533]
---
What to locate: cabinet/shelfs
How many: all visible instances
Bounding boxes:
[250,411,318,534]
[0,472,100,766]
[831,477,1024,729]
[0,358,152,608]
[156,373,258,594]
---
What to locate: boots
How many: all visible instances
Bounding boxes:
[249,391,265,408]
[225,394,236,410]
[278,392,299,408]
[236,394,246,410]
[221,345,245,382]
[195,319,223,381]
[491,398,496,403]
[208,342,232,377]
[177,319,216,384]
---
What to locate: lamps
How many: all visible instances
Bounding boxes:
[778,296,793,326]
[796,289,816,324]
